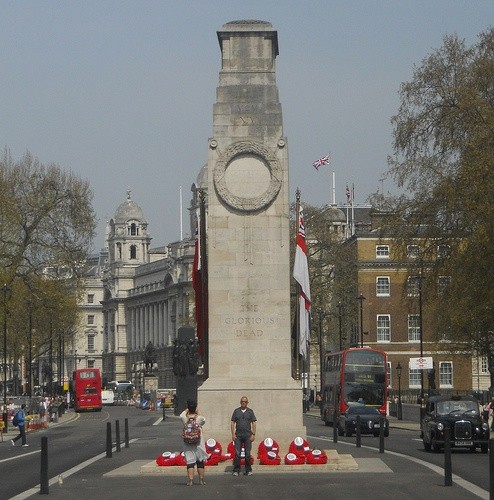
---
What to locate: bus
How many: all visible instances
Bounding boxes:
[105,381,132,405]
[75,369,102,414]
[320,348,388,427]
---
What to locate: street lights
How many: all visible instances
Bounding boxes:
[317,307,332,389]
[25,298,65,400]
[357,295,368,348]
[396,362,402,421]
[336,304,347,352]
[0,282,13,433]
[415,271,426,405]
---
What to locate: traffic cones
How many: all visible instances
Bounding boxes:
[27,413,48,430]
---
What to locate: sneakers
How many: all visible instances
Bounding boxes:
[11,440,15,446]
[22,444,29,447]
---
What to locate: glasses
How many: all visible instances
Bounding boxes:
[242,401,247,403]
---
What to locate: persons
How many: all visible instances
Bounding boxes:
[172,336,202,376]
[427,361,436,388]
[231,396,257,477]
[303,392,310,413]
[142,340,159,376]
[173,394,178,415]
[11,403,30,447]
[479,397,494,432]
[178,398,209,486]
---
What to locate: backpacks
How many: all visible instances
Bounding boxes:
[13,410,21,427]
[183,414,200,444]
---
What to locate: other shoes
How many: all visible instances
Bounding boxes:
[248,472,254,476]
[187,480,193,486]
[200,478,206,485]
[233,472,239,477]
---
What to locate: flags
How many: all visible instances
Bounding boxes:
[292,204,312,362]
[346,185,351,205]
[192,201,208,369]
[311,155,330,171]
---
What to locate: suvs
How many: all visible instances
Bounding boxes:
[421,395,490,454]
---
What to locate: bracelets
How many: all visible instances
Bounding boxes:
[252,433,255,435]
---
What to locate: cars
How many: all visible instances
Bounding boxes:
[135,388,176,410]
[337,406,390,439]
